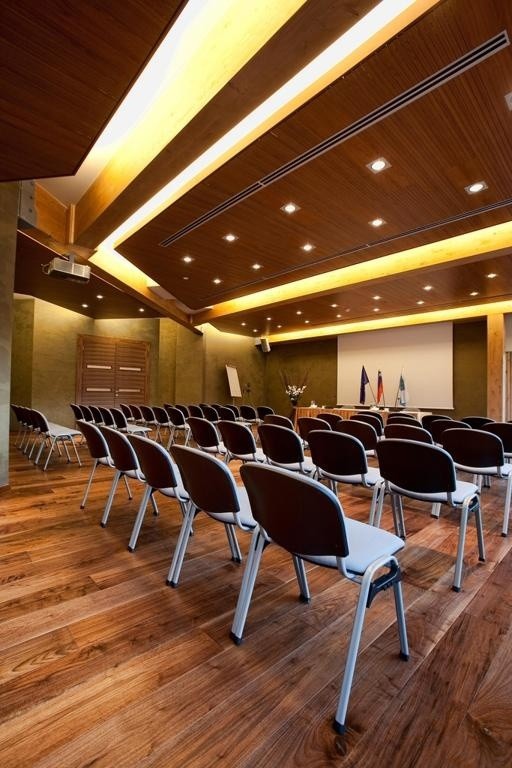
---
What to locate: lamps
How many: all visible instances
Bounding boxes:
[260,336,272,354]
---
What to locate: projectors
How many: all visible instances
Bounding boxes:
[45,259,91,283]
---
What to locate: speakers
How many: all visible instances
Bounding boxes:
[261,339,271,353]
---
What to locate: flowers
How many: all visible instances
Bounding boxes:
[279,368,313,405]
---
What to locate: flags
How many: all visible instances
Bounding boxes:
[376,369,383,405]
[359,364,370,403]
[399,376,406,407]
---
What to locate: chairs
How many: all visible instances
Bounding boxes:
[169,444,306,639]
[373,439,485,593]
[129,434,238,581]
[234,462,410,733]
[70,400,512,541]
[11,403,83,470]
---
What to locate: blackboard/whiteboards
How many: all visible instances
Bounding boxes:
[226,364,242,398]
